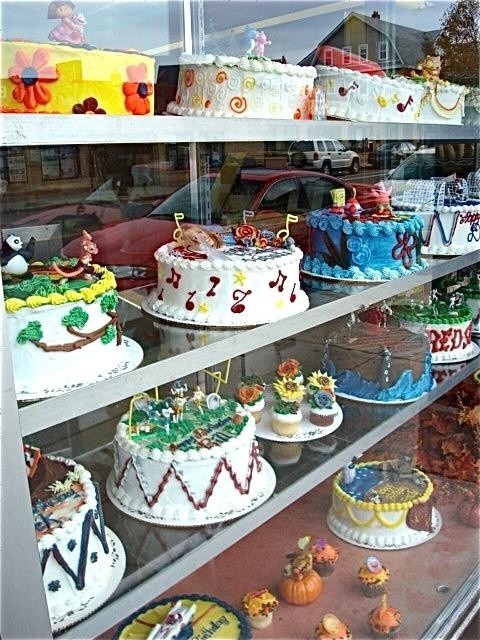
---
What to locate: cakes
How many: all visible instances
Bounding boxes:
[0,0,480,125]
[0,184,479,639]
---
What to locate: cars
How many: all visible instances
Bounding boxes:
[61,169,399,293]
[13,156,256,250]
[378,145,435,206]
[369,141,415,169]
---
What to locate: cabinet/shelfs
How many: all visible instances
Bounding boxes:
[0,0,480,638]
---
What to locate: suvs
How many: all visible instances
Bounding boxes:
[288,143,360,176]
